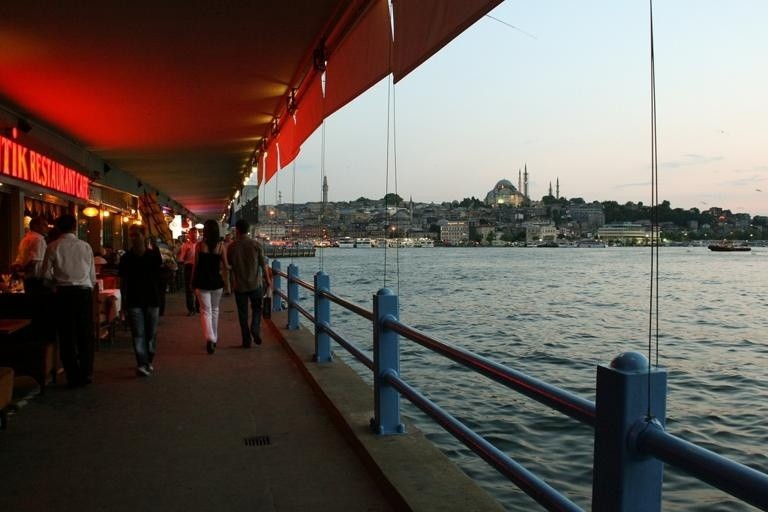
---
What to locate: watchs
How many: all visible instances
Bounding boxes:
[118,224,166,376]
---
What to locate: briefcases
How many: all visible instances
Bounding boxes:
[262,294,272,319]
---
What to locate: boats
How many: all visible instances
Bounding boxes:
[708,244,751,251]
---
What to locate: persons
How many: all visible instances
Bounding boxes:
[43,216,98,386]
[179,219,272,354]
[12,216,53,294]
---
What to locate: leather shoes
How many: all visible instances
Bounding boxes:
[207,341,216,354]
[243,331,262,348]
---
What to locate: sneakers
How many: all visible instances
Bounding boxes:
[137,362,154,376]
